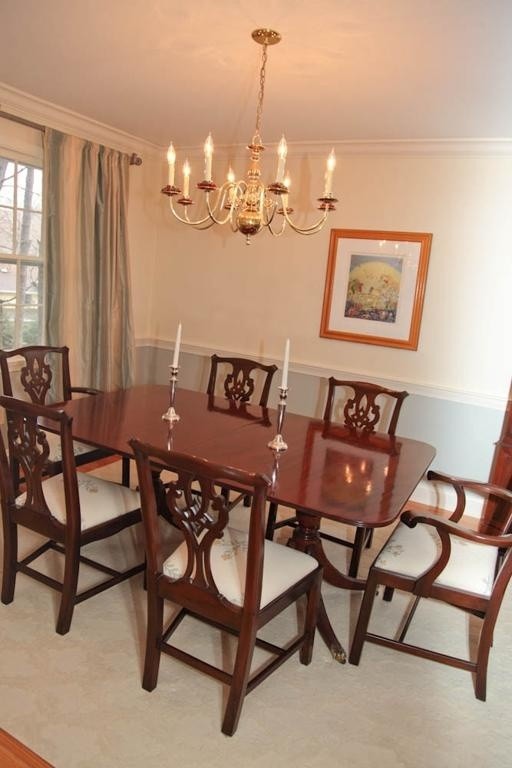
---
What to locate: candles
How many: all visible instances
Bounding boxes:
[172,320,182,364]
[279,338,291,391]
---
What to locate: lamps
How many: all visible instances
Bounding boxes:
[163,25,339,239]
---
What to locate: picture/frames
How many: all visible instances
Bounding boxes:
[315,224,434,351]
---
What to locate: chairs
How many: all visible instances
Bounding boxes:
[0,396,152,637]
[206,353,278,508]
[265,375,409,581]
[2,347,134,495]
[347,465,512,701]
[124,432,323,737]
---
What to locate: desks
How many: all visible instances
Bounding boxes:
[22,386,437,663]
